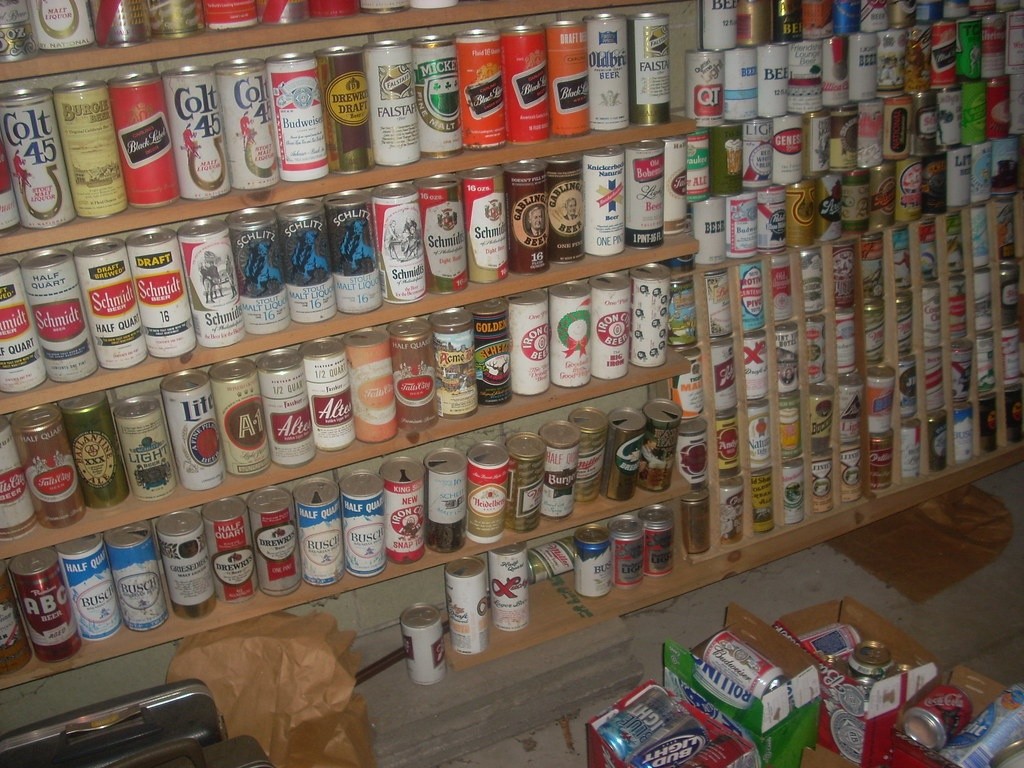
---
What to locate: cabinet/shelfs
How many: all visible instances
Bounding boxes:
[3,0,1024,668]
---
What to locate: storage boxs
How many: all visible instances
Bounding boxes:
[589,595,1016,766]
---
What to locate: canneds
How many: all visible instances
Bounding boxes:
[595,623,1024,768]
[0,0,1024,684]
